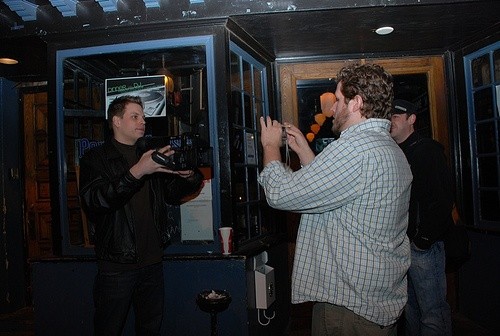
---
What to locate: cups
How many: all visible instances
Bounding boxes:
[218,225,235,255]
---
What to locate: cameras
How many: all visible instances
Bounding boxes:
[281,126,289,141]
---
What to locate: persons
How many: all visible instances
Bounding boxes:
[257,62,414,336]
[387,97,457,334]
[77,96,207,336]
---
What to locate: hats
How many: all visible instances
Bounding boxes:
[390,98,417,114]
